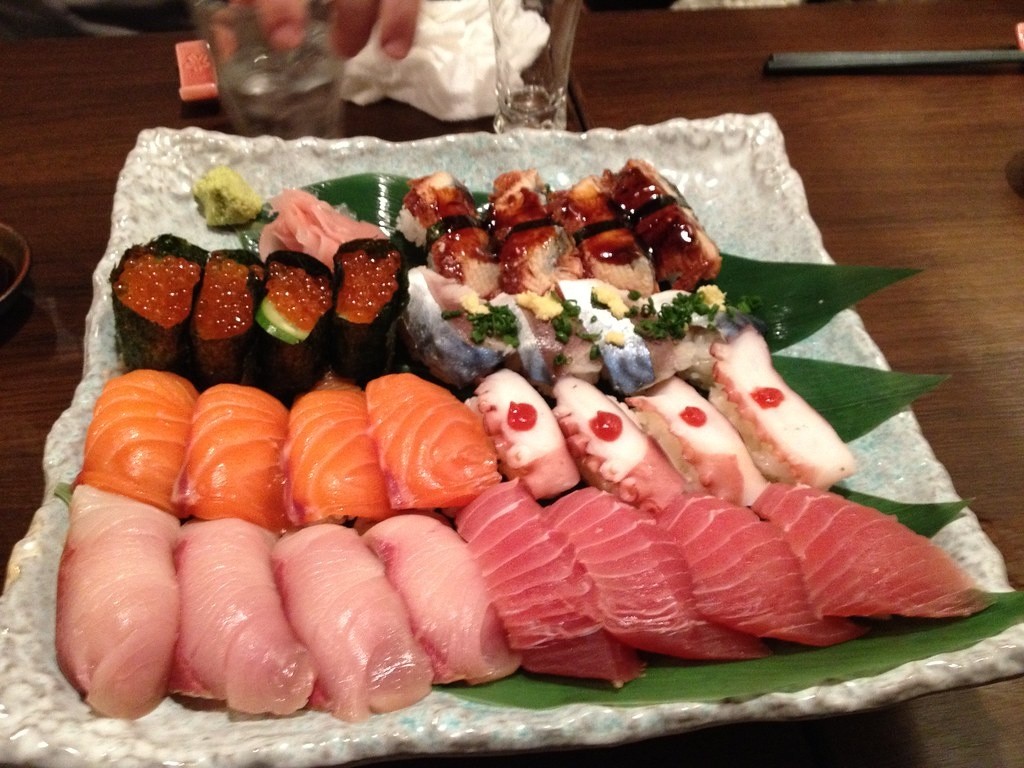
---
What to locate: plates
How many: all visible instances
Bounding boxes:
[0,110,1024,767]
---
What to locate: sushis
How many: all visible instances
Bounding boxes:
[54,155,992,720]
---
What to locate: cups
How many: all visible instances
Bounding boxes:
[192,1,341,139]
[489,1,579,133]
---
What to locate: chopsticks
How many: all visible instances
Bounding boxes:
[766,48,1024,72]
